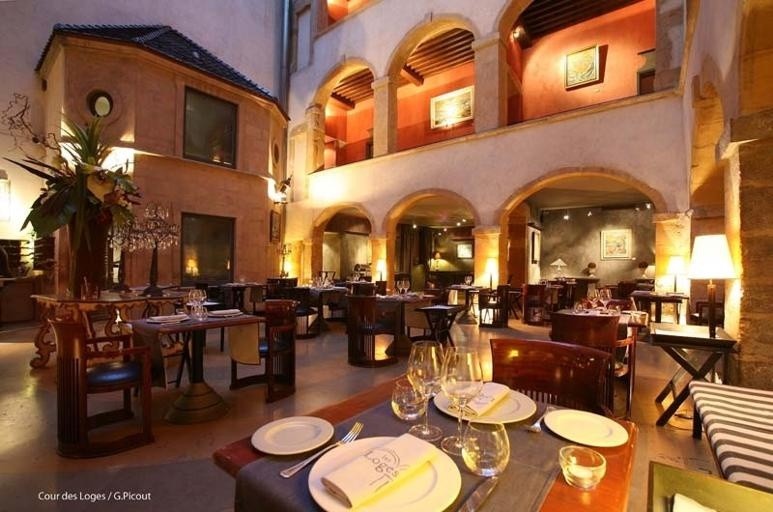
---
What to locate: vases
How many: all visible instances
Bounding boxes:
[110,248,133,293]
[140,240,163,296]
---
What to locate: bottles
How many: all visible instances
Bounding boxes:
[189,306,207,321]
[391,377,426,422]
[462,422,509,477]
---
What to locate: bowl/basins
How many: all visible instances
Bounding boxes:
[557,445,607,491]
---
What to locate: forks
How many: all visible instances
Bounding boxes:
[524,404,555,435]
[280,421,363,480]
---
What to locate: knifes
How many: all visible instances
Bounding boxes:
[455,477,499,512]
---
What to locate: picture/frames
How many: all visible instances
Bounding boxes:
[428,83,474,129]
[454,242,474,259]
[599,228,632,260]
[563,43,600,91]
[531,230,540,263]
[269,208,281,245]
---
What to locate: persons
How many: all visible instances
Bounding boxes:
[581,262,597,278]
[638,261,656,278]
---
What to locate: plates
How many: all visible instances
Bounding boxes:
[251,415,335,456]
[210,311,244,318]
[433,382,537,425]
[150,314,188,324]
[308,435,462,512]
[543,407,629,449]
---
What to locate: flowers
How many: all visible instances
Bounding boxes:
[136,200,179,253]
[109,217,145,252]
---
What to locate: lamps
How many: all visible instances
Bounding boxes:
[665,255,687,293]
[549,257,567,277]
[433,251,441,271]
[687,233,740,338]
[276,172,292,198]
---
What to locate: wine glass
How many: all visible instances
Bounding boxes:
[598,288,613,314]
[406,340,444,441]
[402,281,411,297]
[441,346,483,455]
[395,281,403,295]
[464,276,472,287]
[189,289,207,306]
[587,289,600,310]
